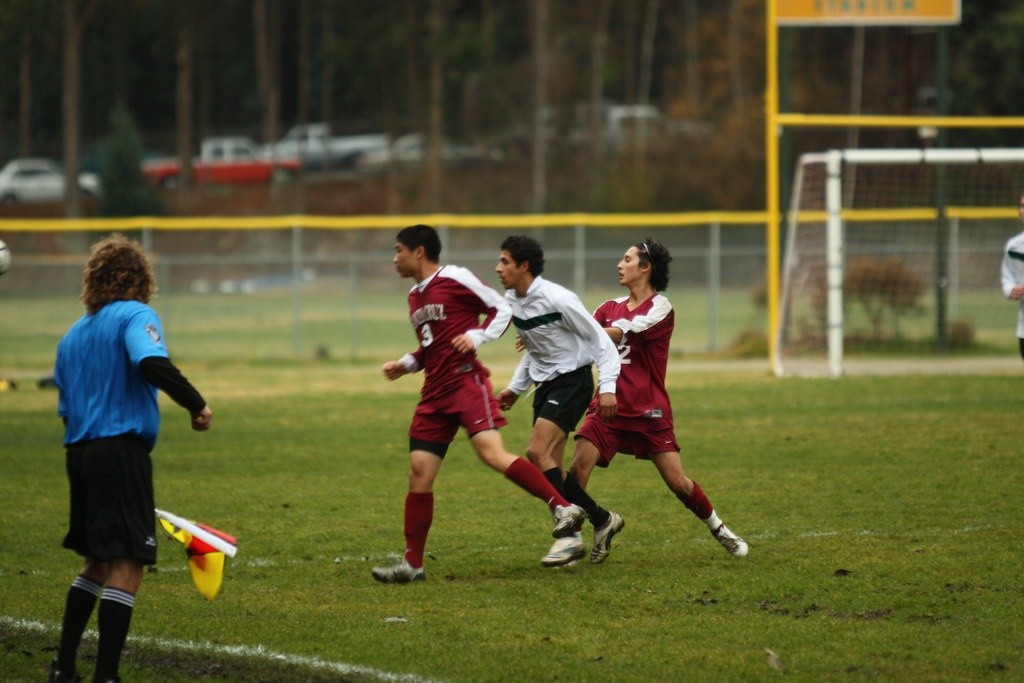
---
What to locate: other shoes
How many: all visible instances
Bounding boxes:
[49,659,79,683]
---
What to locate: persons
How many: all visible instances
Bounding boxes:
[1000,193,1024,360]
[48,238,213,683]
[494,236,625,567]
[371,224,587,583]
[514,237,748,558]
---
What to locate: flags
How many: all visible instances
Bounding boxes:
[160,514,237,602]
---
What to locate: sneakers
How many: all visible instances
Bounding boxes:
[590,509,624,564]
[542,529,587,567]
[370,555,427,584]
[552,503,585,537]
[711,522,748,558]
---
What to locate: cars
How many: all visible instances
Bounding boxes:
[0,157,103,213]
[136,136,303,190]
[481,98,719,177]
[278,123,391,171]
